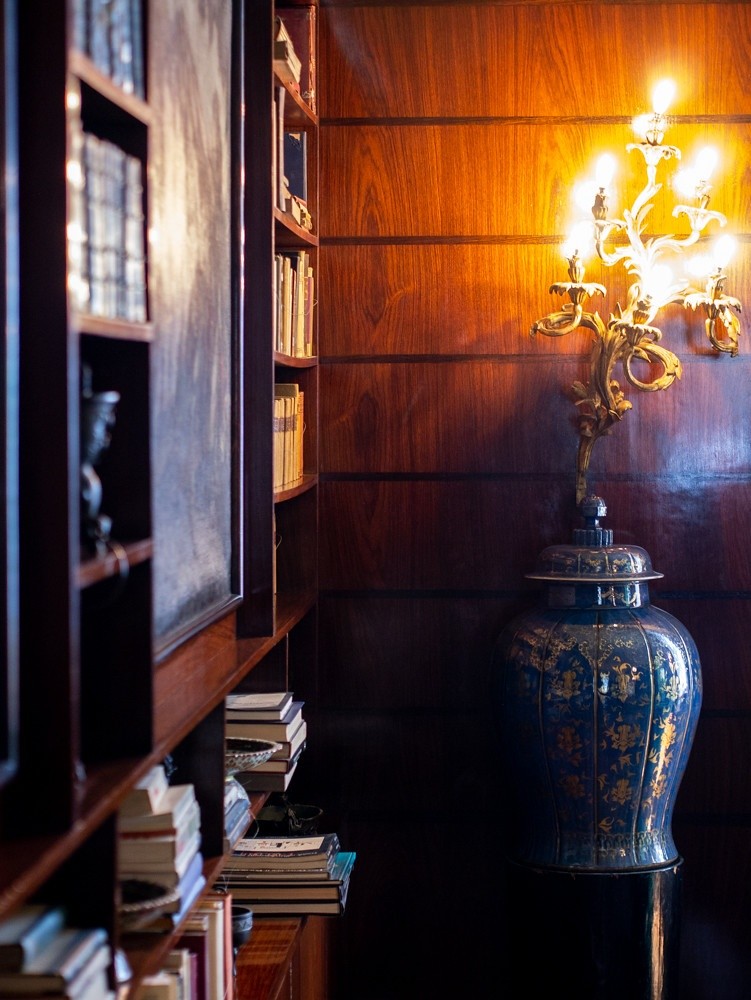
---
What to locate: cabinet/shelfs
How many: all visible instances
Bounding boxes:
[0,0,356,1000]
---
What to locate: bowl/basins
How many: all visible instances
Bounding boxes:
[232,906,253,948]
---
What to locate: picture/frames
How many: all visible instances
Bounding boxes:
[150,1,248,673]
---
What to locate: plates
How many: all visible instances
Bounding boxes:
[224,735,283,775]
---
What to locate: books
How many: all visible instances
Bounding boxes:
[74,3,151,323]
[273,17,314,495]
[0,692,356,999]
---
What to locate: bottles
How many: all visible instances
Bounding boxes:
[502,491,704,871]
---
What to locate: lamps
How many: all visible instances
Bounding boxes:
[530,77,741,497]
